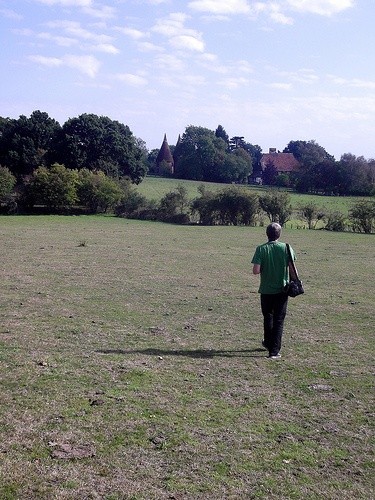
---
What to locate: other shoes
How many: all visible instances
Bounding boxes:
[261,338,280,359]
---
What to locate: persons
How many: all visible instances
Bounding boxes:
[251,223,301,359]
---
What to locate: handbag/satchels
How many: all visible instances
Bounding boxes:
[284,243,304,298]
